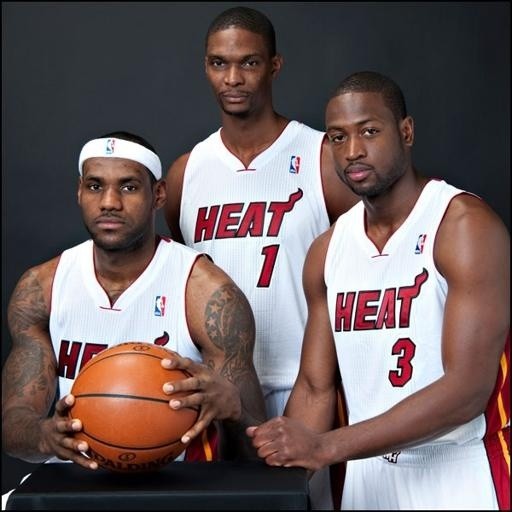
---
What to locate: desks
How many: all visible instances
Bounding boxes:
[7,463,311,512]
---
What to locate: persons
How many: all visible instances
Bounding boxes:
[164,6,349,512]
[0,131,267,512]
[246,70,511,510]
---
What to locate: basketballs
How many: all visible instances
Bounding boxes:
[67,343,203,474]
